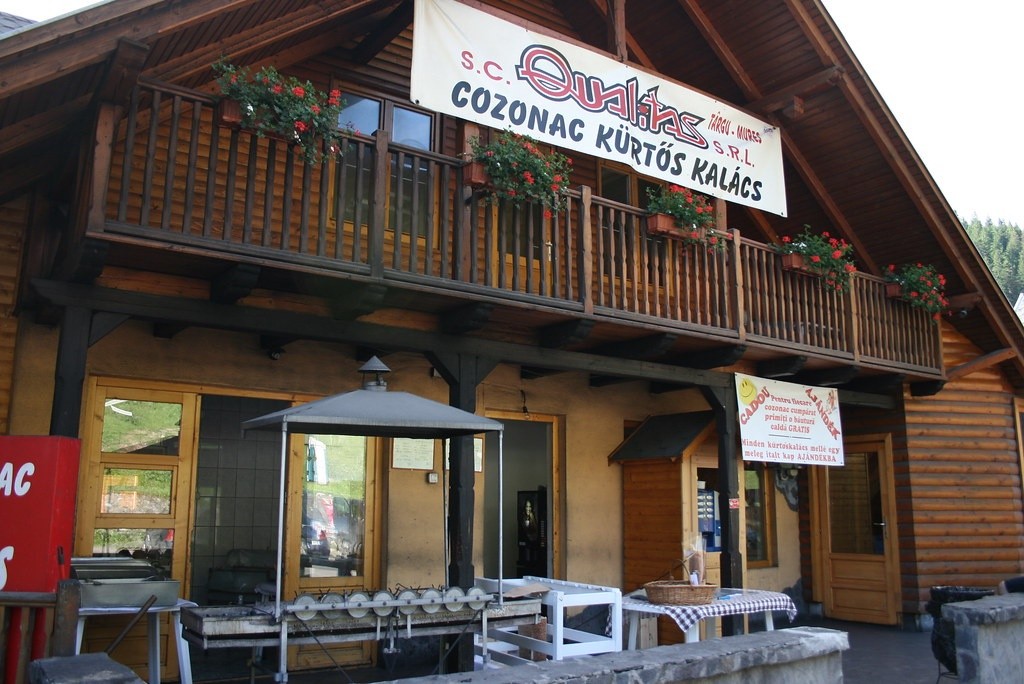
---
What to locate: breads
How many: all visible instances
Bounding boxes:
[689,550,707,586]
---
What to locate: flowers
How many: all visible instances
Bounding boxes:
[768,224,859,295]
[211,54,361,170]
[645,184,733,256]
[456,124,573,220]
[880,261,952,325]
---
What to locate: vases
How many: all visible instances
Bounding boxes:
[645,213,706,246]
[216,97,294,144]
[779,252,820,277]
[885,282,902,298]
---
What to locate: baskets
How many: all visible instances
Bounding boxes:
[643,559,718,605]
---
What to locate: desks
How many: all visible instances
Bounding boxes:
[605,588,798,650]
